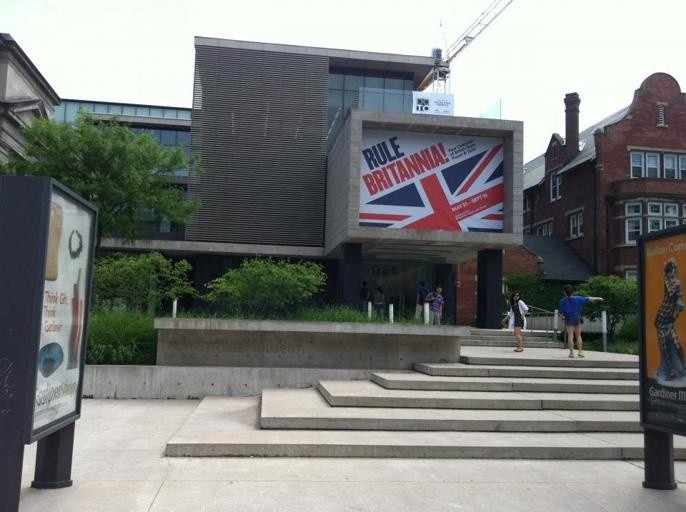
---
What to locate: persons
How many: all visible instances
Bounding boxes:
[558,285,606,358]
[374,286,385,316]
[501,291,529,352]
[414,281,431,321]
[360,281,372,312]
[654,259,686,382]
[424,286,445,327]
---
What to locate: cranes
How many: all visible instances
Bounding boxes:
[416,0,514,93]
